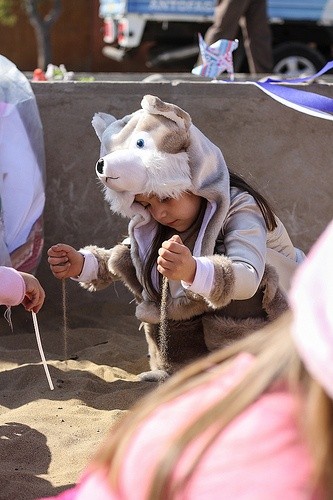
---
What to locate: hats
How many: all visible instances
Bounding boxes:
[91,95,232,323]
[286,219,333,396]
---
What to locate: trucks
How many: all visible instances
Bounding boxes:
[99,0,333,76]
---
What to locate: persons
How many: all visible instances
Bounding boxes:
[47,95,306,381]
[0,265,47,314]
[32,217,333,500]
[193,0,274,74]
